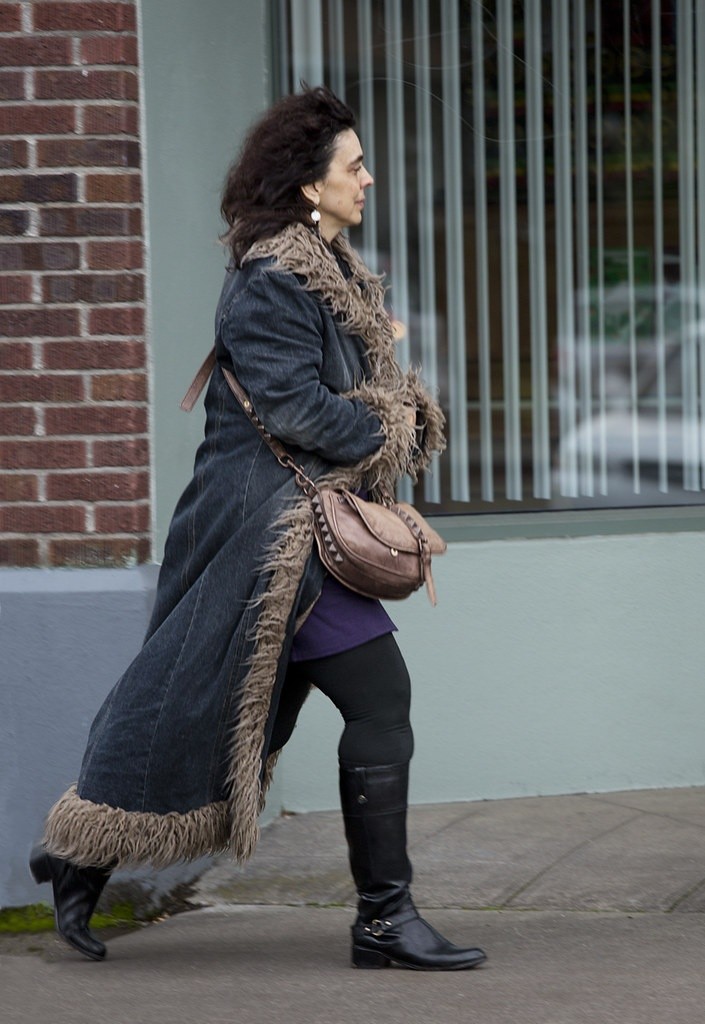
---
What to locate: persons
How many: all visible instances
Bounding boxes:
[27,89,486,973]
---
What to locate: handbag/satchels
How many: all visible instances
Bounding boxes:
[306,488,449,602]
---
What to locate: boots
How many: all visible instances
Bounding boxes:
[338,759,488,972]
[29,837,120,960]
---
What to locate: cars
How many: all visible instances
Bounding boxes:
[552,319,705,503]
